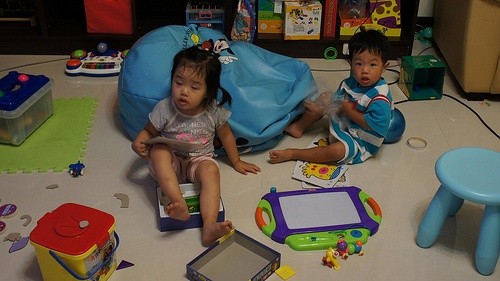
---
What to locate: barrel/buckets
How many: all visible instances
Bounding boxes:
[29,203,119,281]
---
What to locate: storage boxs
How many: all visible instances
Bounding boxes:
[0,71,54,145]
[186,229,282,281]
[186,0,402,42]
[29,202,120,281]
[397,53,447,100]
[155,183,225,231]
[83,0,133,34]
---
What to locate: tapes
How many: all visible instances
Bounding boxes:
[406,135,429,151]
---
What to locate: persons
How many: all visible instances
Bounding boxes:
[131,46,262,249]
[269,30,394,164]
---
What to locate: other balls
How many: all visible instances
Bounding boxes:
[383,107,406,145]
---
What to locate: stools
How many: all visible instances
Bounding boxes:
[413,146,500,274]
